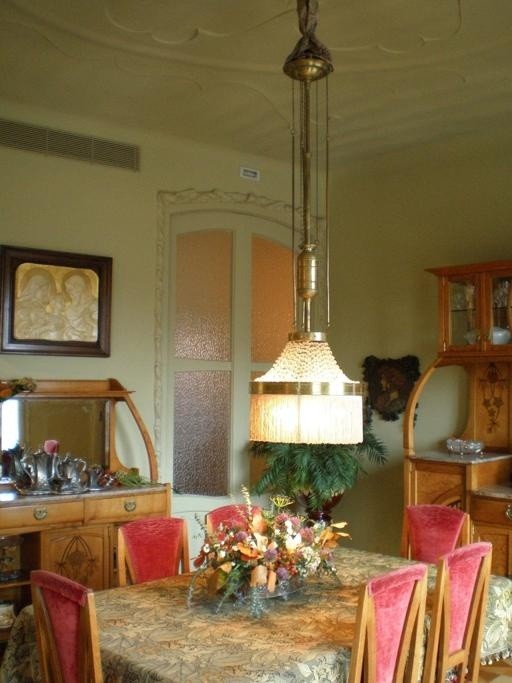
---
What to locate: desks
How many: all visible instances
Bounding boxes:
[0,542,512,682]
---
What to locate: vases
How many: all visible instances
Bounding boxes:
[237,561,309,602]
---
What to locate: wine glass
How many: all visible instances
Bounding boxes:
[492,281,508,307]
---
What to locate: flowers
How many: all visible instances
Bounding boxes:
[186,490,355,616]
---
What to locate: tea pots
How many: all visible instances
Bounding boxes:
[13,444,107,497]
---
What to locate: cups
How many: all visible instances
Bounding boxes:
[1,604,17,630]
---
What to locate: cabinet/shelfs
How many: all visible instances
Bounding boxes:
[0,483,171,651]
[400,262,511,574]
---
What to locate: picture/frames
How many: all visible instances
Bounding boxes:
[1,242,112,358]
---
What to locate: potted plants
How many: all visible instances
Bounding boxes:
[249,390,388,509]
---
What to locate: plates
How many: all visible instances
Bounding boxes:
[446,438,486,456]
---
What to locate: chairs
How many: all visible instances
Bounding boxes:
[116,517,191,587]
[401,503,474,563]
[350,563,427,682]
[205,505,264,568]
[30,569,103,682]
[423,541,492,683]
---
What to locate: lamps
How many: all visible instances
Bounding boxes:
[245,3,368,449]
